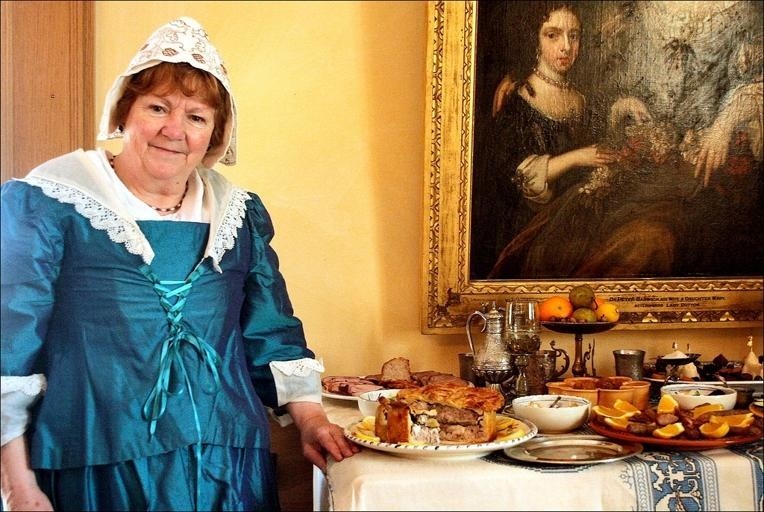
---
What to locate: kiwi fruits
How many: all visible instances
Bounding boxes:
[568,285,595,307]
[572,308,597,321]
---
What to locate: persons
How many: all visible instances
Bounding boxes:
[0,18,361,512]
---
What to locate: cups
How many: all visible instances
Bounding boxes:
[613,349,646,380]
[549,374,651,411]
[539,335,569,385]
[458,353,474,383]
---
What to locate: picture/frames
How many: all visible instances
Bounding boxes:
[419,1,763,336]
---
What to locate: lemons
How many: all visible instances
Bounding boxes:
[597,304,619,321]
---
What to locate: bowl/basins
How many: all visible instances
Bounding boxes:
[658,383,738,411]
[356,386,407,415]
[509,393,592,436]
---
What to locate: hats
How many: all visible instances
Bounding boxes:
[101,18,238,166]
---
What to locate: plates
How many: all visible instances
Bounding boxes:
[749,400,763,418]
[656,353,700,364]
[343,410,534,462]
[587,419,762,451]
[315,373,377,401]
[506,435,641,467]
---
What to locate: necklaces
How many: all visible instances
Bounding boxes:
[108,154,189,212]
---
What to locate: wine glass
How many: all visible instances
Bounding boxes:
[503,301,541,397]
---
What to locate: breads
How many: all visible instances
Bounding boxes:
[370,383,507,444]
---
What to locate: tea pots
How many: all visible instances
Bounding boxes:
[465,301,513,394]
